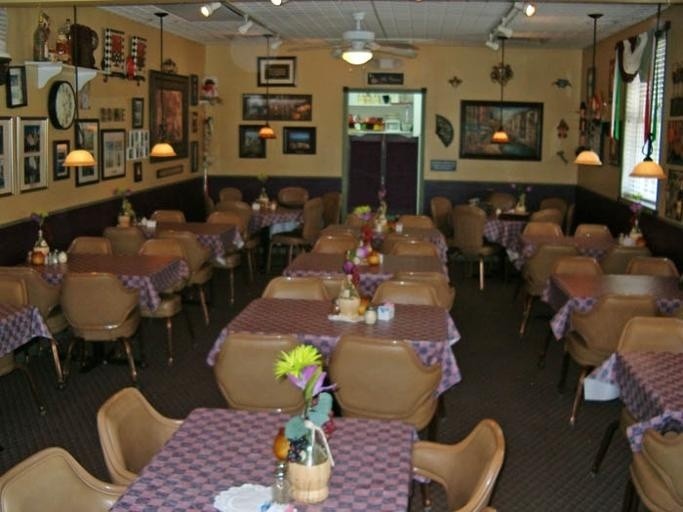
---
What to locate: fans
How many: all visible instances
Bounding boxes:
[287,12,420,58]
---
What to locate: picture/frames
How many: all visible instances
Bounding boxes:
[586,66,596,102]
[664,66,683,223]
[238,56,316,158]
[459,100,544,160]
[0,5,199,198]
[590,121,603,162]
[607,120,623,167]
[608,58,615,105]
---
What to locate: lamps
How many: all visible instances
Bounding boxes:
[491,36,510,144]
[341,49,373,65]
[629,4,666,179]
[573,13,604,166]
[200,2,286,51]
[270,0,290,6]
[485,1,535,50]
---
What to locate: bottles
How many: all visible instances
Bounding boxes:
[270,201,278,212]
[328,294,395,324]
[26,248,68,266]
[33,18,72,64]
[619,233,632,247]
[273,464,292,504]
[675,179,683,221]
[128,216,147,227]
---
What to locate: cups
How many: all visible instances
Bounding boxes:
[382,96,390,103]
[392,222,404,235]
[636,237,645,248]
[273,427,291,460]
[146,219,157,233]
